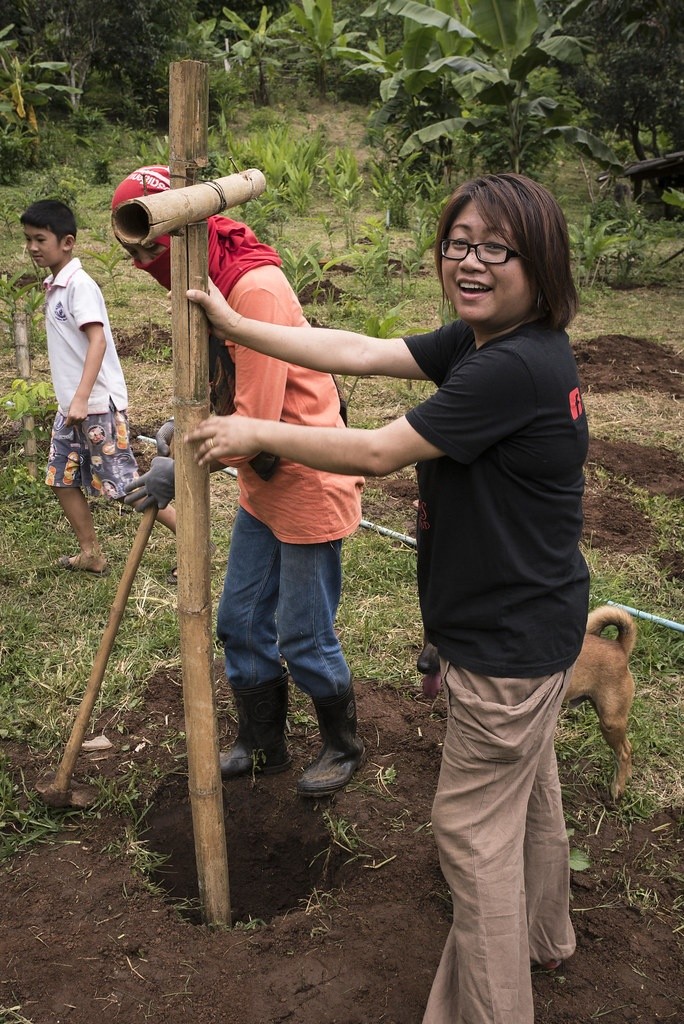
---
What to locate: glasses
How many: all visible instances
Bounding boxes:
[441,238,523,264]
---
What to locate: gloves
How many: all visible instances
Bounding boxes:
[124,455,176,513]
[156,415,176,457]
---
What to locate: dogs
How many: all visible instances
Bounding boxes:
[418,606,637,798]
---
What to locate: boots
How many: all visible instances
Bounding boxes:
[220,667,292,778]
[298,677,366,796]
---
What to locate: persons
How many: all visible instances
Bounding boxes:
[166,174,590,1024]
[20,200,216,583]
[112,164,365,798]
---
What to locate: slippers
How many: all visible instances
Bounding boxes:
[167,564,180,585]
[51,555,110,577]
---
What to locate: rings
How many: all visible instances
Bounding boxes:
[210,438,214,448]
